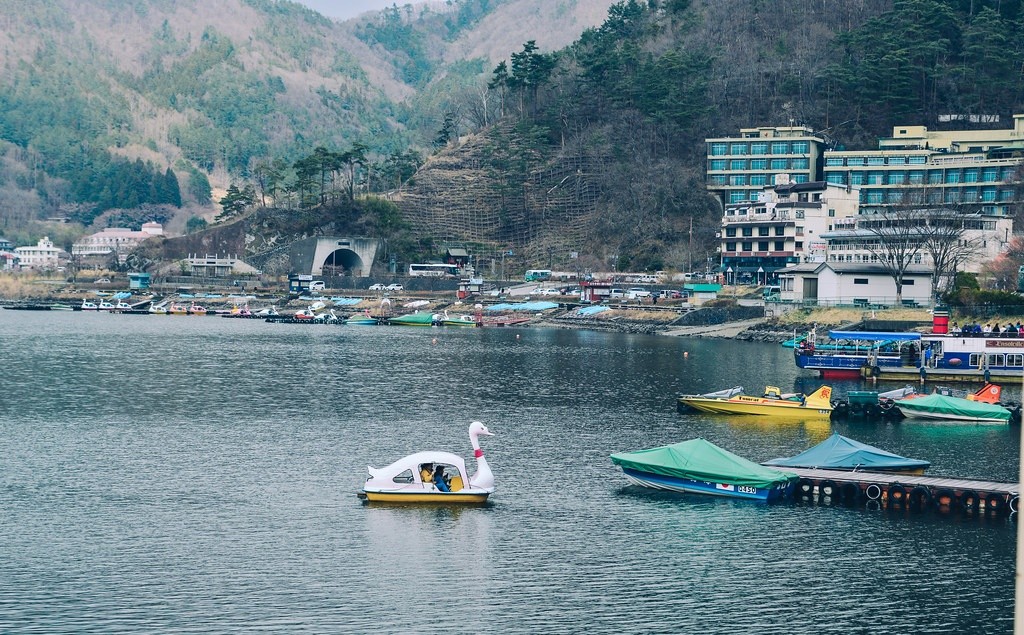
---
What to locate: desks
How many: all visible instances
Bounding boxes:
[909,302,919,308]
[874,304,883,309]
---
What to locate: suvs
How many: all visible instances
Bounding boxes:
[628,288,649,297]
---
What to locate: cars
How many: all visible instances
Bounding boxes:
[309,281,402,292]
[766,293,780,301]
[529,269,716,292]
[94,279,110,285]
[672,291,688,298]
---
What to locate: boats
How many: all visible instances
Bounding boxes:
[678,385,838,421]
[3,298,478,327]
[763,431,926,498]
[358,421,498,504]
[892,392,1012,424]
[611,437,798,504]
[878,381,1001,404]
[782,304,1024,384]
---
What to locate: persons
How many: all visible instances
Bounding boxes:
[652,296,657,305]
[952,321,1024,338]
[420,463,434,482]
[501,287,511,294]
[432,466,450,492]
[638,295,641,304]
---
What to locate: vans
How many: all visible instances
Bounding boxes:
[609,289,627,298]
[659,290,678,298]
[762,286,779,299]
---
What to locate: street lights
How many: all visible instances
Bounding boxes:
[332,239,348,276]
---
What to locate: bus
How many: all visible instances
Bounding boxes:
[410,263,461,280]
[525,271,551,281]
